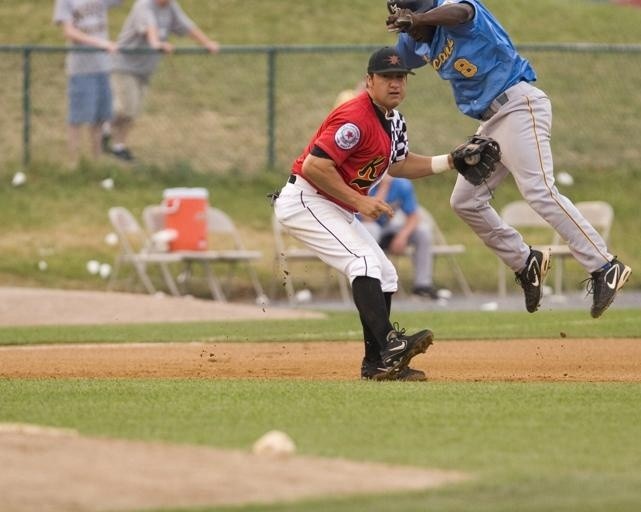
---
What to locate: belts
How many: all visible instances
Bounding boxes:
[480,93,509,121]
[288,175,296,183]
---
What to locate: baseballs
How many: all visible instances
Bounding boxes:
[560,171,573,183]
[87,259,98,275]
[100,264,110,277]
[254,432,292,462]
[12,172,25,188]
[439,288,450,298]
[297,290,311,303]
[102,179,114,191]
[35,261,49,272]
[105,234,119,246]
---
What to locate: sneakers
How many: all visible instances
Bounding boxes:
[380,329,435,375]
[591,257,632,319]
[411,284,440,301]
[361,356,426,381]
[515,247,552,314]
[101,133,134,161]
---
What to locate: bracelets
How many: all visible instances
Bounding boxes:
[431,154,452,174]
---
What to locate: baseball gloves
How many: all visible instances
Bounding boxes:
[450,135,502,185]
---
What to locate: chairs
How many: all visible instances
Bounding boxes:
[107,198,618,306]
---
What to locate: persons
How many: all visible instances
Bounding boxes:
[356,172,450,299]
[53,0,221,173]
[271,48,501,383]
[385,2,632,319]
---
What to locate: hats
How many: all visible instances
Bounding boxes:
[367,46,416,77]
[387,0,434,16]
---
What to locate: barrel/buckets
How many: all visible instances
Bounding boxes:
[160,187,208,252]
